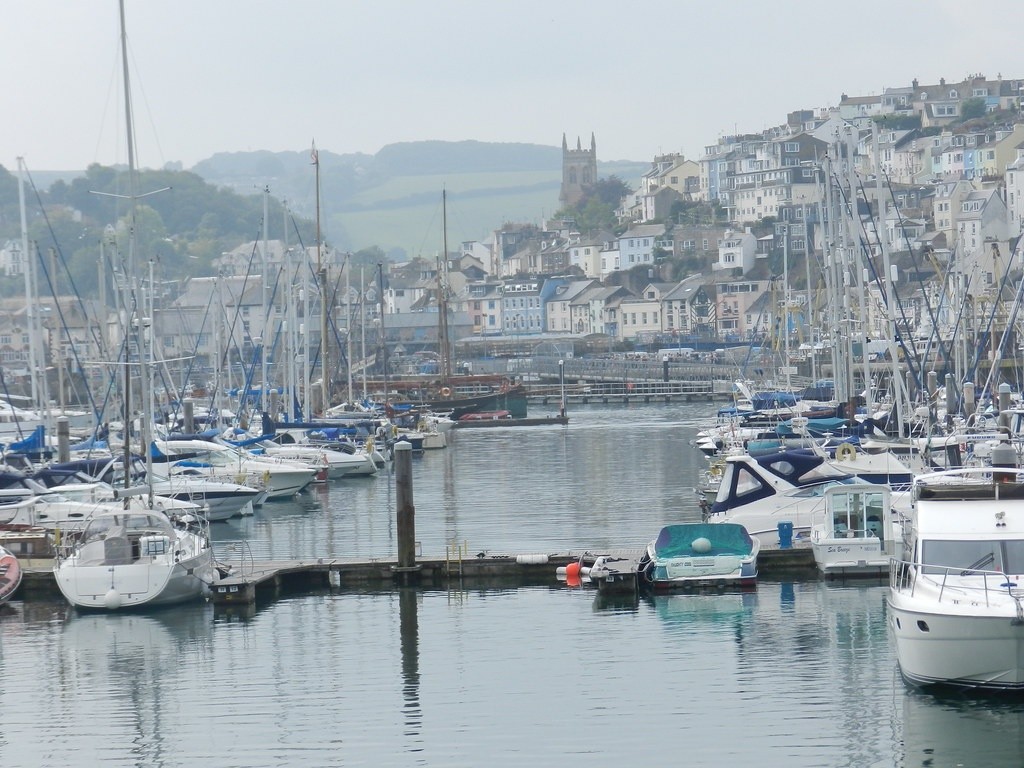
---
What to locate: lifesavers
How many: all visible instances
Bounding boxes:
[263,470,270,481]
[368,438,372,452]
[392,425,397,436]
[836,442,856,461]
[442,387,451,397]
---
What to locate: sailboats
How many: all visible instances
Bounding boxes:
[0,0,454,617]
[342,184,528,417]
[696,113,1024,549]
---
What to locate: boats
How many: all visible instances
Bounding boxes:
[461,408,513,420]
[811,481,905,575]
[887,433,1024,695]
[641,523,759,589]
[589,556,641,590]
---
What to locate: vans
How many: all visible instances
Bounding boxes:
[659,348,695,357]
